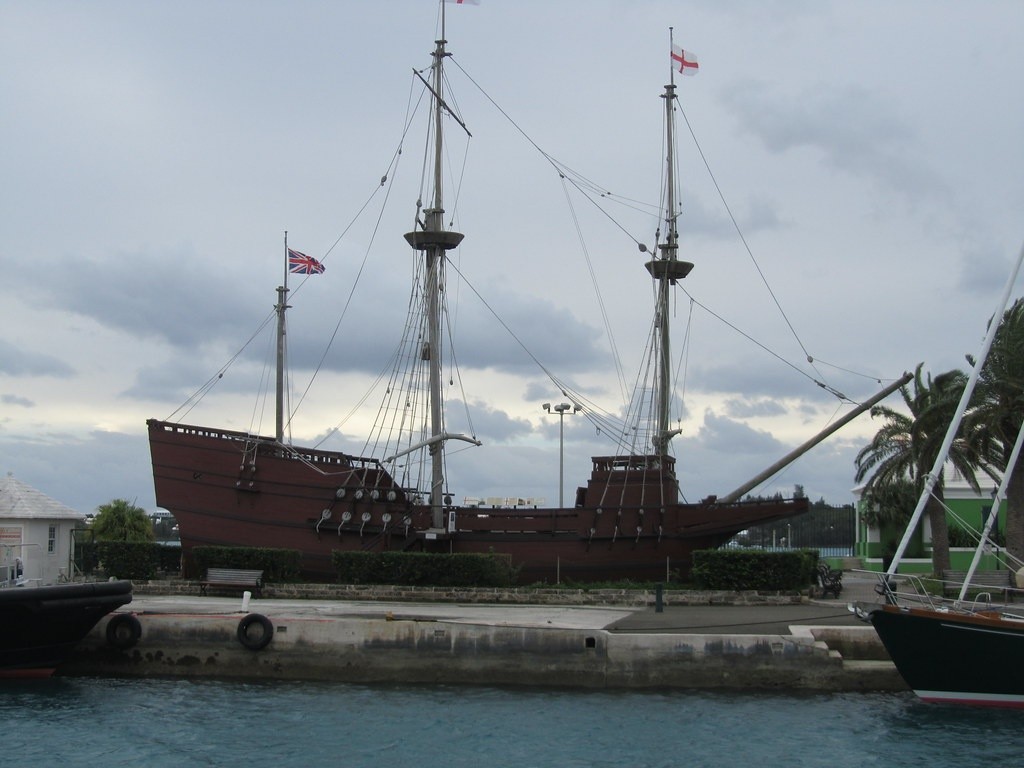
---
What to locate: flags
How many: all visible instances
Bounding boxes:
[672,44,699,76]
[289,249,325,274]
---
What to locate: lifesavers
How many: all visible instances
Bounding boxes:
[106,612,142,649]
[237,611,276,649]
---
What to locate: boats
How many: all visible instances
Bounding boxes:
[852,237,1024,709]
[0,544,132,681]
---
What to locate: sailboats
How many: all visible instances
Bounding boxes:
[145,37,916,583]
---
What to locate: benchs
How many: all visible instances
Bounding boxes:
[942,569,1015,603]
[188,568,265,600]
[816,560,844,599]
